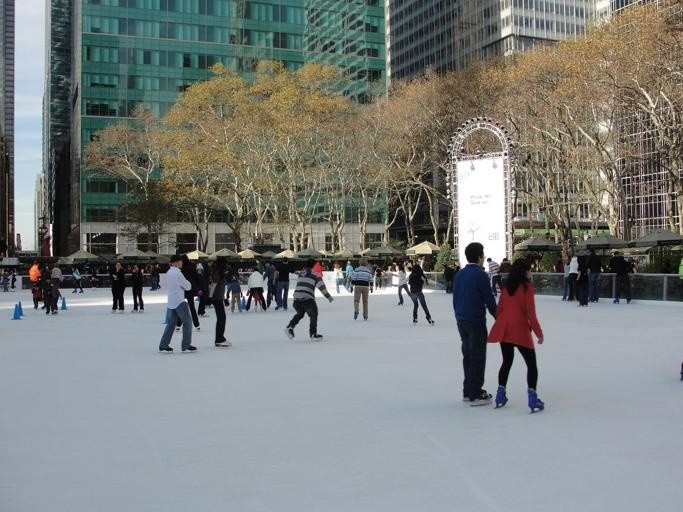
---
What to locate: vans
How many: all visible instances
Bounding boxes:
[606,254,650,272]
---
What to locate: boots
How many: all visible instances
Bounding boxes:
[529,388,543,408]
[496,386,508,403]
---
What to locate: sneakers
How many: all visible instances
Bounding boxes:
[464,390,492,401]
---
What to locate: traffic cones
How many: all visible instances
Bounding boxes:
[240,296,247,309]
[18,301,25,317]
[60,296,67,311]
[9,303,23,321]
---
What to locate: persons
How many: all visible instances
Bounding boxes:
[488,258,512,296]
[175,254,307,346]
[372,263,399,290]
[445,240,492,408]
[492,257,549,415]
[5,259,172,293]
[563,251,602,306]
[159,255,196,351]
[285,260,333,341]
[131,265,144,311]
[111,263,125,313]
[29,259,61,314]
[313,261,355,293]
[532,264,563,293]
[351,260,374,321]
[442,265,460,293]
[610,251,632,303]
[398,265,412,304]
[408,265,435,326]
